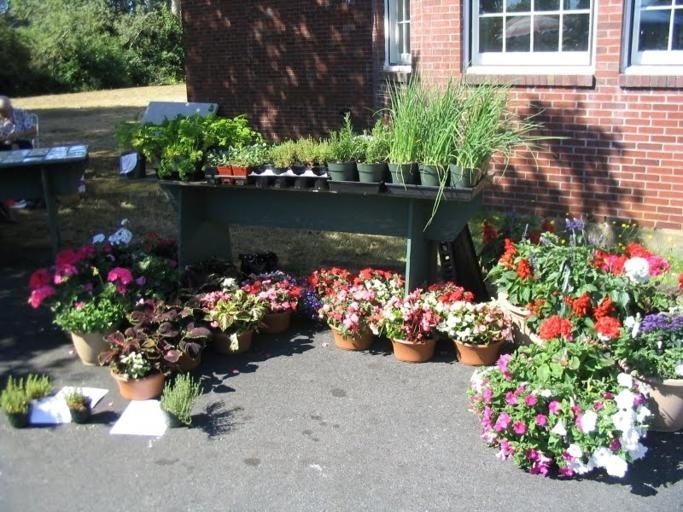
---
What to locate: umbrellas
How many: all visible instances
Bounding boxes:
[497,16,566,40]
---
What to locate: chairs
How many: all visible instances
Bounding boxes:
[28,114,40,148]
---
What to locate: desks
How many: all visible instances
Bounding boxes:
[118,175,492,303]
[0,143,90,265]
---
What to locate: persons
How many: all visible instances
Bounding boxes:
[0,96,37,150]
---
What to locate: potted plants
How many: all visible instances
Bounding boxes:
[0,374,30,429]
